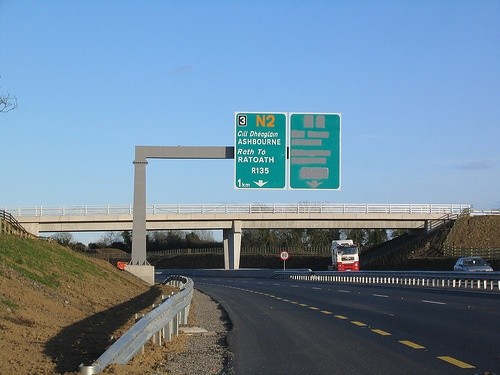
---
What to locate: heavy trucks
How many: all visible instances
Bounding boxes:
[327,238,359,271]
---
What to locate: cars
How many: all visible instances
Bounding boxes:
[453,256,493,272]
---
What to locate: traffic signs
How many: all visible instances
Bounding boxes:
[236,113,288,189]
